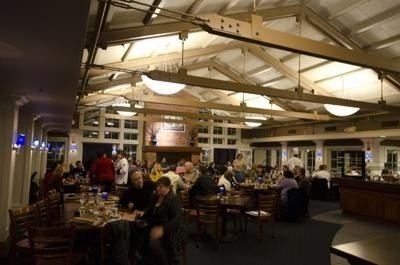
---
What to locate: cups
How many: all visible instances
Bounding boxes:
[102,192,108,201]
[112,208,118,217]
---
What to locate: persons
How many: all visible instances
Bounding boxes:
[174,152,246,223]
[313,164,330,189]
[248,153,307,202]
[30,150,183,265]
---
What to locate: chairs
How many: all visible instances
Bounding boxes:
[0,163,400,264]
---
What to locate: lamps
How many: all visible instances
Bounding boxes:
[281,148,372,162]
[111,14,361,128]
[13,133,78,154]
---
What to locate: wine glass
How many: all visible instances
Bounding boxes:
[80,193,87,208]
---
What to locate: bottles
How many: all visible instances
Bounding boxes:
[258,177,262,184]
[220,187,224,194]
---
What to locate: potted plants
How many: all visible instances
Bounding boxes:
[186,128,199,147]
[146,127,160,146]
